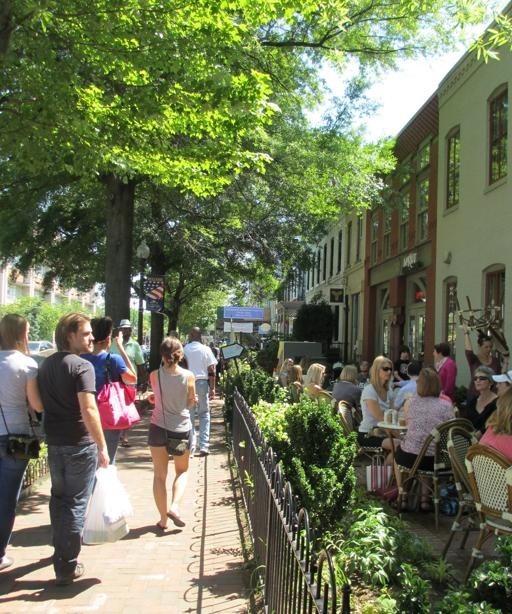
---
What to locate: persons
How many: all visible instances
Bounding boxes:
[148,336,195,530]
[111,318,145,447]
[79,315,137,464]
[280,347,451,486]
[463,327,511,509]
[0,313,44,565]
[39,313,111,585]
[183,326,218,458]
[433,343,457,399]
[389,369,457,509]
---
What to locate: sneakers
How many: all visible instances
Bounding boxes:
[200,447,209,454]
[57,563,83,584]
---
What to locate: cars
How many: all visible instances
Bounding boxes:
[24,340,56,359]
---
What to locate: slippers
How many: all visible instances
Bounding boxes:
[157,512,185,529]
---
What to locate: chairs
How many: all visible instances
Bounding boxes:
[462,444,512,587]
[395,418,476,531]
[441,427,479,562]
[335,400,396,494]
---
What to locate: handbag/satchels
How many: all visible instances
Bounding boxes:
[83,465,130,544]
[366,455,393,492]
[8,436,39,460]
[167,437,189,454]
[97,353,140,430]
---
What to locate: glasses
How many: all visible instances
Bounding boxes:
[473,376,488,380]
[383,367,391,371]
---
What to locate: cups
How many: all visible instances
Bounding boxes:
[383,408,405,426]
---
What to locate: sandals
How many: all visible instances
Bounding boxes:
[391,498,434,514]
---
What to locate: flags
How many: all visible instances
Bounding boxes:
[143,274,164,314]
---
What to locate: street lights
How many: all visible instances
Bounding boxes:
[136,237,150,345]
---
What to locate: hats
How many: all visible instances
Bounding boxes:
[116,320,130,327]
[492,370,512,384]
